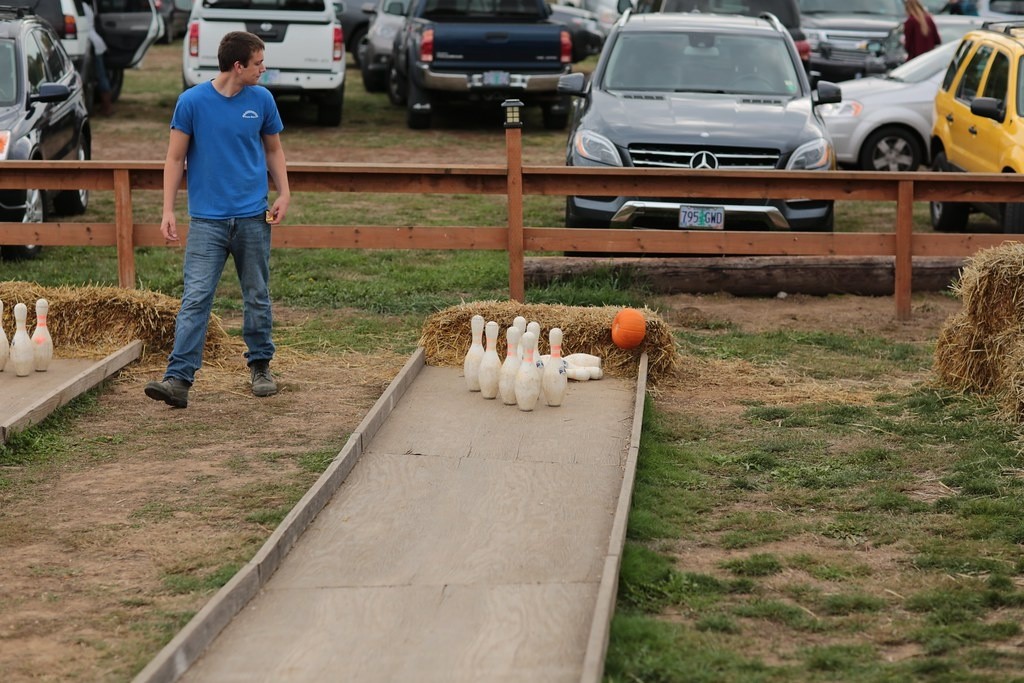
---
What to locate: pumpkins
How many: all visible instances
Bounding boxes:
[612,308,646,347]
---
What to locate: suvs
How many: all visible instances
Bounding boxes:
[929,16,1024,237]
[0,6,91,253]
[558,4,838,256]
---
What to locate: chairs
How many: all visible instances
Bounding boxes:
[436,0,457,10]
[499,0,520,13]
[388,2,404,14]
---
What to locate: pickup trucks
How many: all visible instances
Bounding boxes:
[386,0,576,129]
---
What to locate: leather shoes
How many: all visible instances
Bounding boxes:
[250,368,278,397]
[144,378,190,408]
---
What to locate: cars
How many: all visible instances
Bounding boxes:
[814,30,961,171]
[0,0,1024,112]
[183,2,349,128]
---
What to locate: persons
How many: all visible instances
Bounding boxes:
[143,31,290,408]
[902,0,942,61]
